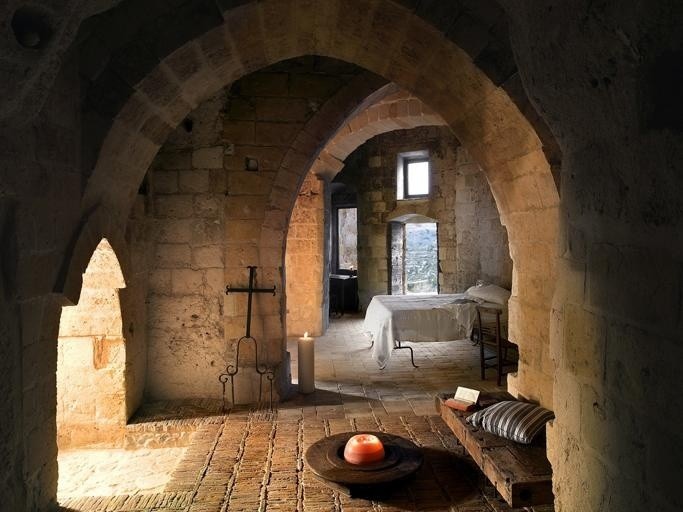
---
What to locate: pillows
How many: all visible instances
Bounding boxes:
[465,281,511,305]
[466,401,556,446]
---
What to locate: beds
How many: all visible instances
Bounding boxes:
[361,294,513,370]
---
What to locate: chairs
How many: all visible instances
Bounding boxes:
[474,306,513,386]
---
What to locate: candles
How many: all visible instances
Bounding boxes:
[297,331,315,395]
